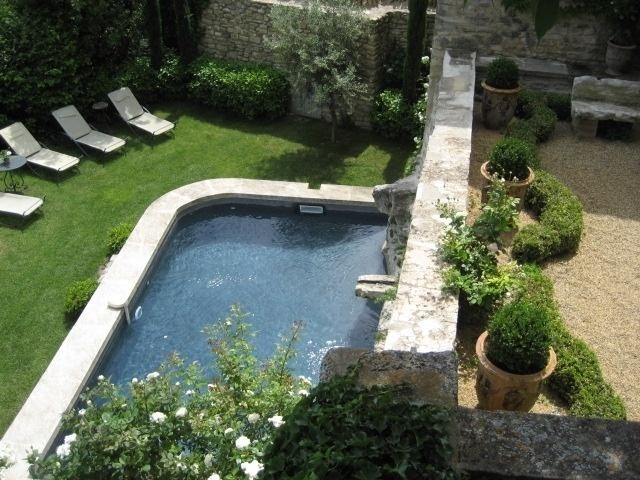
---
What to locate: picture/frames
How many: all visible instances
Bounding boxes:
[92,102,108,129]
[0,155,27,194]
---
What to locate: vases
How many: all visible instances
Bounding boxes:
[607,0,639,45]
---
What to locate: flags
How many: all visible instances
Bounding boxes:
[605,40,637,75]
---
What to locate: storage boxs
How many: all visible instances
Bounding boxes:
[570,75,640,140]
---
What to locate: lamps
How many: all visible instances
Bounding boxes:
[0,191,44,229]
[0,122,81,184]
[52,104,127,168]
[107,86,175,142]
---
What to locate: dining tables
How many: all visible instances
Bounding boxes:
[475,279,558,412]
[481,56,521,130]
[0,150,10,163]
[480,137,535,217]
